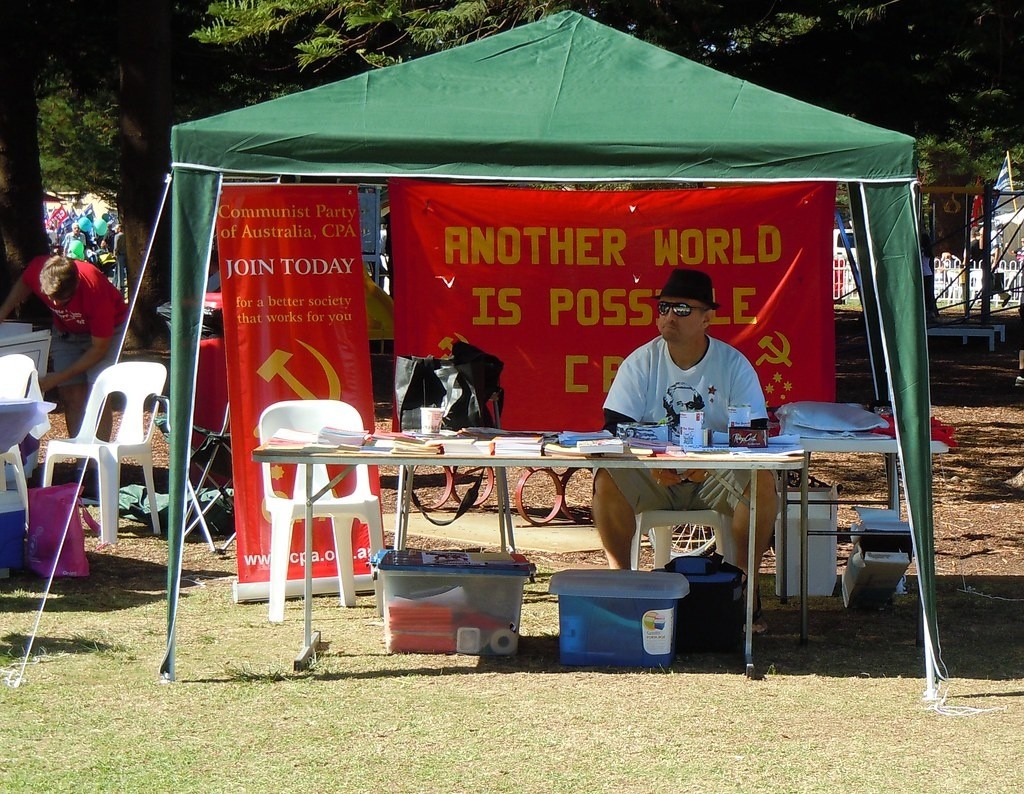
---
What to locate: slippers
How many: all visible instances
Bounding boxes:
[741,594,767,636]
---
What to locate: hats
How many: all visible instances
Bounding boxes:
[650,269,720,310]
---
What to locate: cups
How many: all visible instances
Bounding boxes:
[728,404,751,442]
[680,411,703,446]
[420,408,444,434]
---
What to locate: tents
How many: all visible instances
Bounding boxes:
[155,14,950,709]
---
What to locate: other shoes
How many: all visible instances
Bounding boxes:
[1002,295,1011,307]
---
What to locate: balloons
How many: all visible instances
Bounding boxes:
[94,218,107,236]
[78,217,92,233]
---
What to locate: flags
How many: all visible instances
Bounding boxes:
[970,176,984,237]
[992,155,1013,193]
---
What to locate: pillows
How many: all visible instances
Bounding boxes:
[774,402,891,430]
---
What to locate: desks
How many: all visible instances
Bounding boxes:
[779,404,949,605]
[250,430,806,678]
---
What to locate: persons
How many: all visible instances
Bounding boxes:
[1015,261,1024,388]
[43,220,127,296]
[919,231,943,322]
[966,225,1024,308]
[1,253,130,497]
[590,268,780,619]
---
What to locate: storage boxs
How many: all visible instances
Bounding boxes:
[0,490,25,569]
[549,570,690,668]
[372,550,538,654]
[774,481,838,597]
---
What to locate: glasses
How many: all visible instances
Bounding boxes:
[657,301,709,317]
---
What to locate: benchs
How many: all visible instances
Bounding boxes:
[926,324,995,350]
[930,319,1005,342]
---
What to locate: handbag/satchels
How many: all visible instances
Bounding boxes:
[991,268,1004,294]
[395,341,505,435]
[652,553,748,650]
[27,483,101,578]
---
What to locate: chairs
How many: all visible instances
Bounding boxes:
[631,509,736,570]
[394,388,515,554]
[260,399,384,623]
[43,362,168,544]
